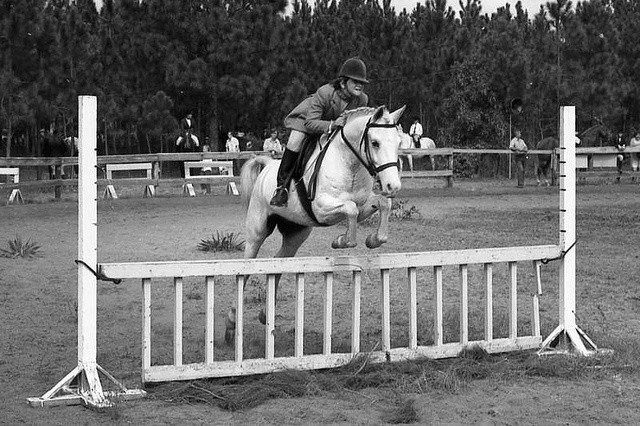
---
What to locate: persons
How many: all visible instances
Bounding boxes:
[244,132,259,151]
[614,131,626,174]
[200,144,212,196]
[269,56,370,207]
[262,128,282,152]
[410,116,423,148]
[174,111,200,153]
[509,130,528,188]
[225,132,241,176]
[630,133,640,172]
[234,131,253,151]
[44,127,69,178]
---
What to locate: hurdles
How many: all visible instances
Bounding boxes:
[24,94,616,413]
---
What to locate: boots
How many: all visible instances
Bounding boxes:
[270,147,299,206]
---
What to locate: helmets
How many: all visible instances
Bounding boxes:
[339,58,369,83]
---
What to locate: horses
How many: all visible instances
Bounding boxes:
[395,122,439,173]
[224,103,409,346]
[176,126,203,195]
[533,119,613,186]
